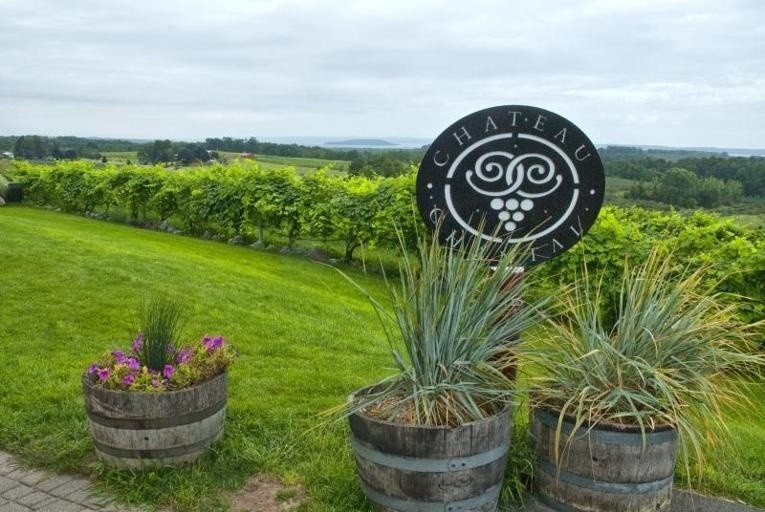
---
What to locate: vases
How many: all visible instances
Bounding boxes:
[80,366,231,471]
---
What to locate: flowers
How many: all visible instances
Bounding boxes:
[88,294,240,393]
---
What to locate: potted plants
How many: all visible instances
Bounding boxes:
[520,223,765,512]
[286,194,580,511]
